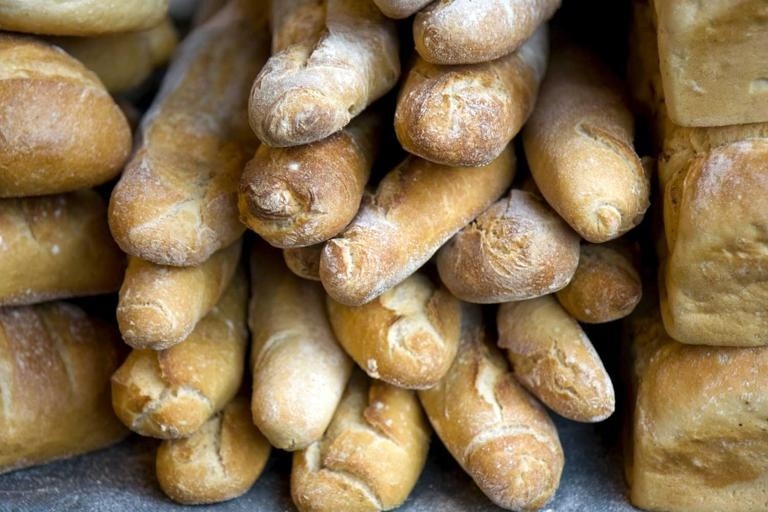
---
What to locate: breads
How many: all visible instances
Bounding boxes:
[0,0,767,512]
